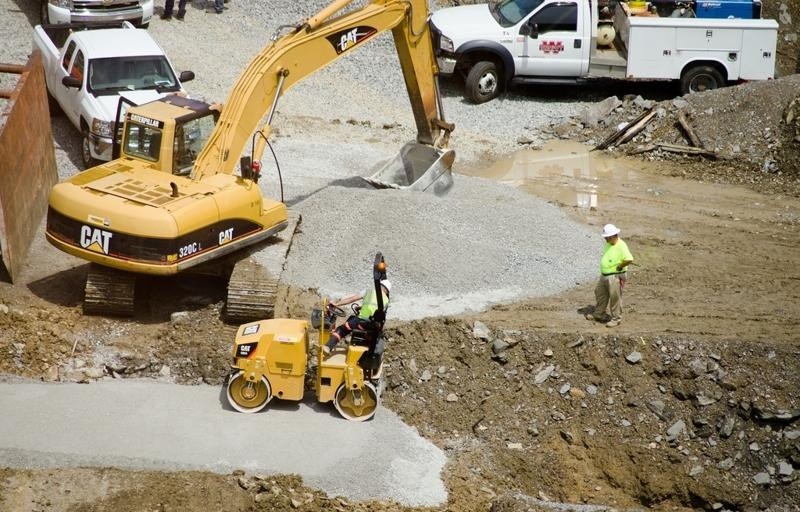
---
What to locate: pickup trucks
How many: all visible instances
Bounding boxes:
[426,0,780,107]
[29,20,204,166]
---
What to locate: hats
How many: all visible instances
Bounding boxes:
[379,279,392,292]
[599,223,622,239]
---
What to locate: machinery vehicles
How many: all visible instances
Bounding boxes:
[43,1,459,326]
[227,292,387,419]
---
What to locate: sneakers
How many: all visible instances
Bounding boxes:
[605,319,621,328]
[585,313,606,321]
[312,342,331,357]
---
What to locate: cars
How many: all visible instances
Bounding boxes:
[40,0,154,32]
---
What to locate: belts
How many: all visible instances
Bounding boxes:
[599,270,627,277]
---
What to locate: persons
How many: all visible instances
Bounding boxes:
[160,0,188,20]
[311,278,392,356]
[149,122,164,158]
[585,222,634,327]
[215,0,230,14]
